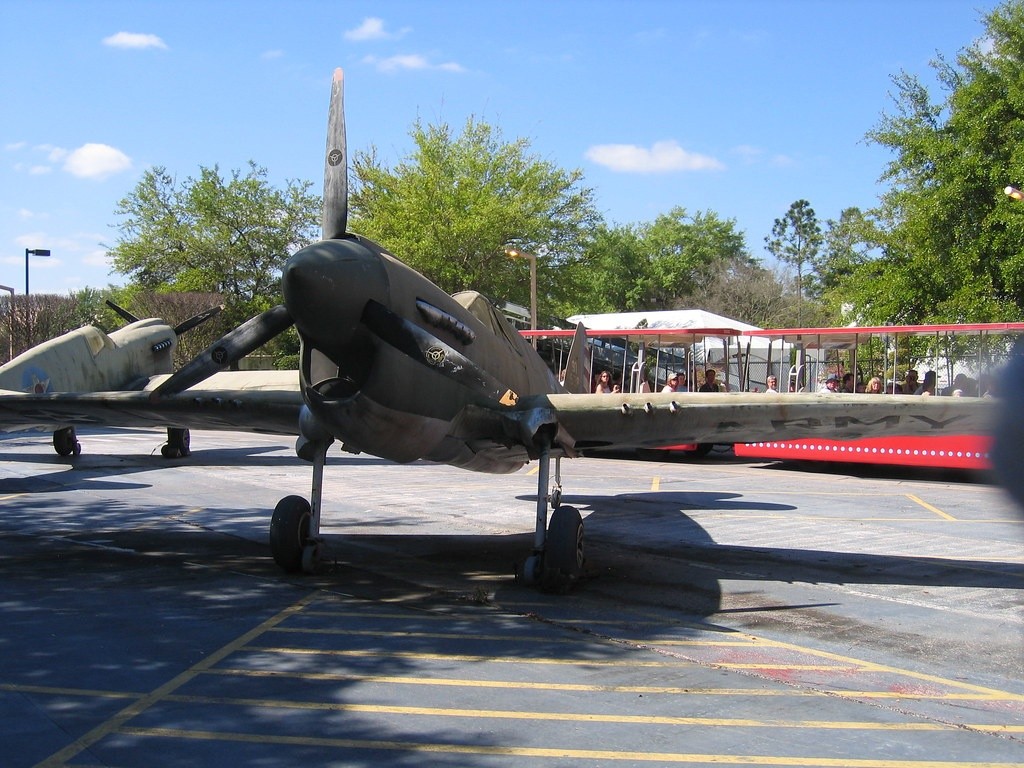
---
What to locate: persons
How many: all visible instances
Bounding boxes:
[559,368,719,393]
[766,370,997,399]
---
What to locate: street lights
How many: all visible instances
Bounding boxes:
[26,248,51,346]
[504,249,538,351]
[1,284,15,360]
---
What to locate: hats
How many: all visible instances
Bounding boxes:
[824,373,841,385]
[668,374,678,380]
[924,370,942,379]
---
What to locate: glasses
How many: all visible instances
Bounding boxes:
[604,375,609,378]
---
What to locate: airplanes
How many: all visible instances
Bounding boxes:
[1,297,227,458]
[0,59,1002,597]
